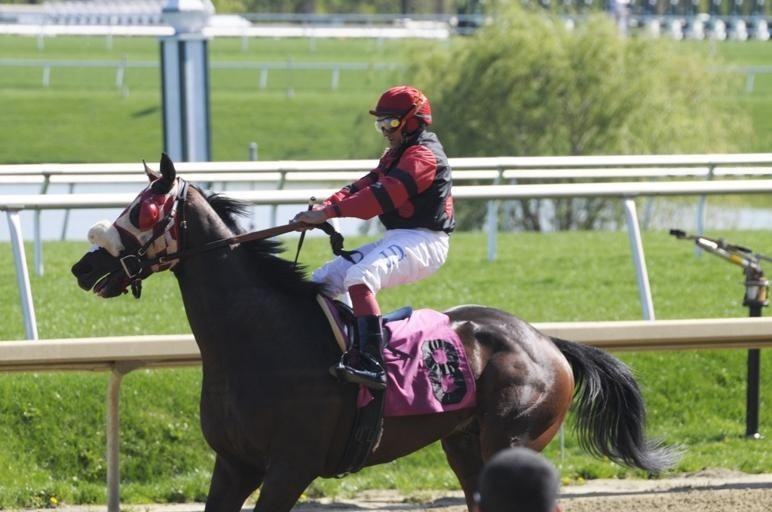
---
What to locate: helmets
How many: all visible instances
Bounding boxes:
[368,86,432,142]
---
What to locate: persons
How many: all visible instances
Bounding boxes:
[294,86,455,390]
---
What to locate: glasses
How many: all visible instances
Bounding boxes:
[373,117,402,134]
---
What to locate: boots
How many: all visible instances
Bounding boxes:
[335,313,388,392]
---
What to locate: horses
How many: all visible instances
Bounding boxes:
[70,150,689,512]
